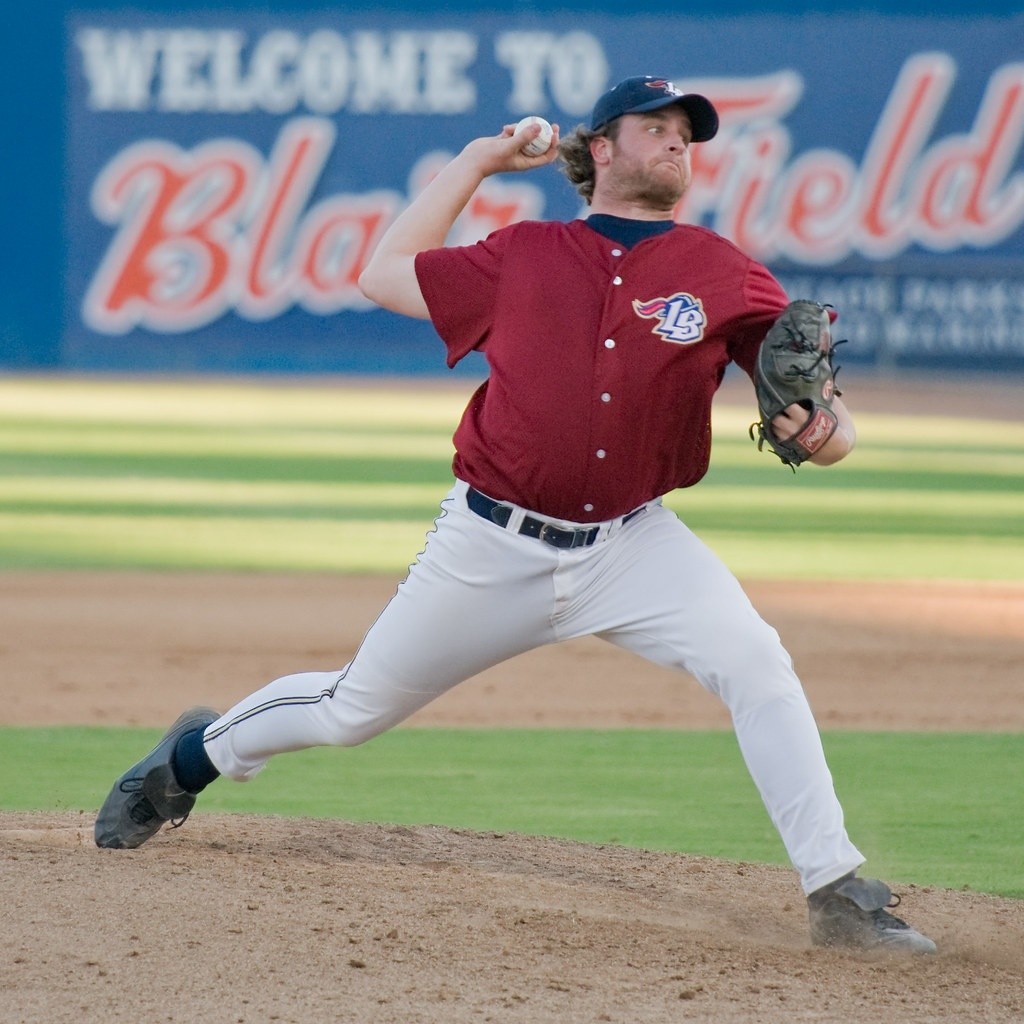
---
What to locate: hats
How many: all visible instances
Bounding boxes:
[591,75,719,143]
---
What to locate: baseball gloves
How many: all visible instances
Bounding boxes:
[752,300,836,468]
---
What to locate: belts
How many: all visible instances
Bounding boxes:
[465,488,645,550]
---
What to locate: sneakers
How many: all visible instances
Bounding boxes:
[94,707,222,850]
[809,877,936,955]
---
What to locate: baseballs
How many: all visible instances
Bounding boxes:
[515,116,554,158]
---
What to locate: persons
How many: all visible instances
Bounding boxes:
[97,77,937,959]
